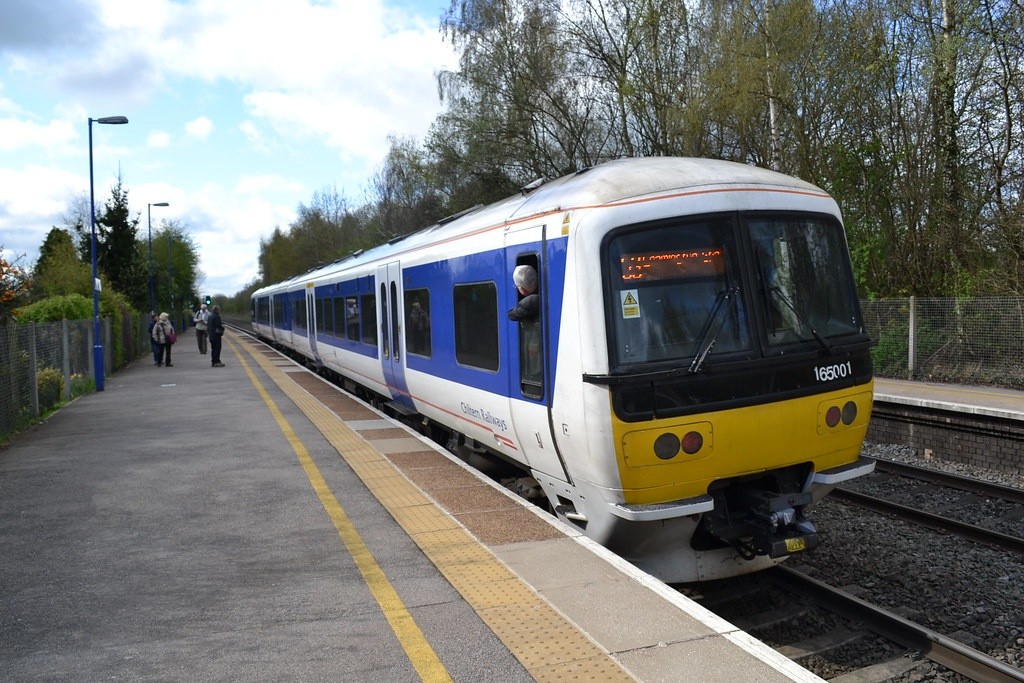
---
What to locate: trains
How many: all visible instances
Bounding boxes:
[249,156,877,583]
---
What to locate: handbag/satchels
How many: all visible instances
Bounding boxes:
[214,327,223,336]
[165,333,177,343]
[193,322,197,326]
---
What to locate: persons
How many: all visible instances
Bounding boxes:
[347,299,358,339]
[148,312,174,367]
[207,304,225,367]
[193,304,211,354]
[409,302,429,353]
[507,265,539,320]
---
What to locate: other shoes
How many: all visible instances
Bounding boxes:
[154,361,164,367]
[200,351,206,354]
[212,362,225,368]
[166,365,173,367]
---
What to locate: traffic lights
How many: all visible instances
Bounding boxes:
[206,297,211,305]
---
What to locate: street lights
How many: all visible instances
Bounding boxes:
[147,203,171,313]
[88,117,129,391]
